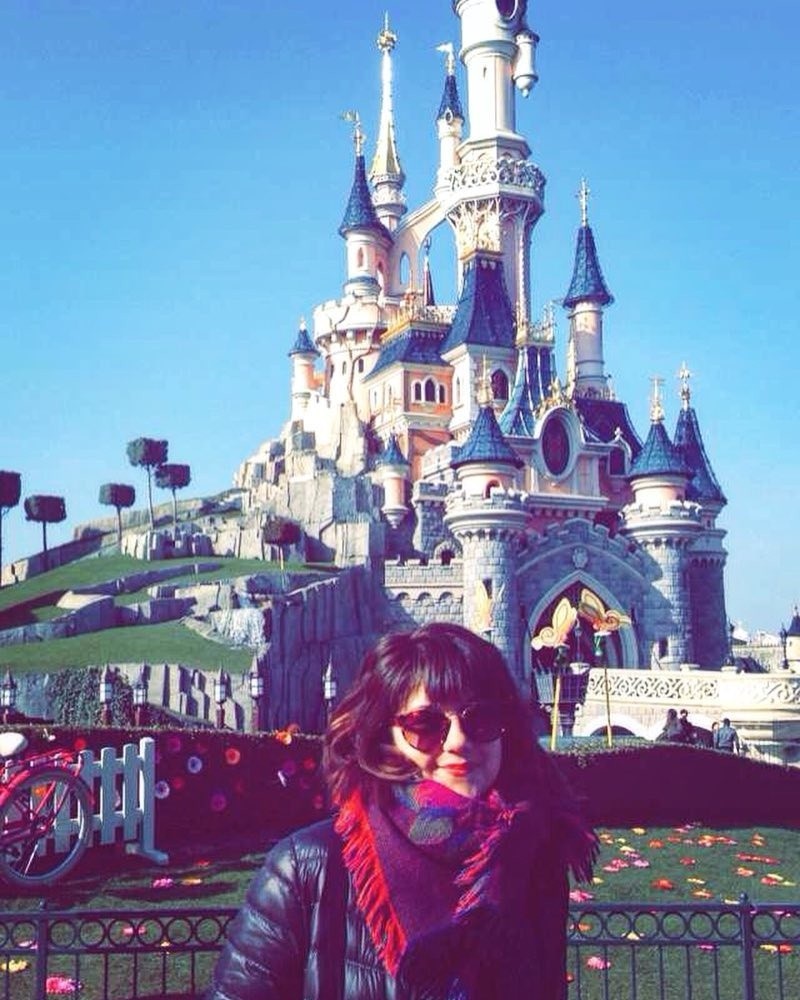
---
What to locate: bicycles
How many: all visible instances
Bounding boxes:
[0,733,96,888]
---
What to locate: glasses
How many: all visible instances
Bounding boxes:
[386,701,506,752]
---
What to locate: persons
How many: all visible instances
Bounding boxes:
[206,622,601,1000]
[710,718,744,757]
[662,708,697,742]
